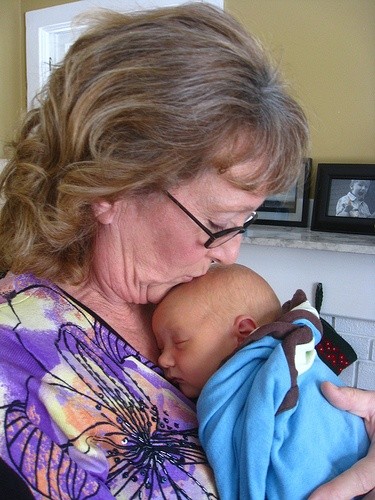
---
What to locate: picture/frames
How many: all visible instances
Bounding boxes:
[310,163,375,236]
[252,158,313,228]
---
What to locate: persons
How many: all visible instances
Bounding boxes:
[336,179,371,218]
[152,262,370,500]
[0,3,375,500]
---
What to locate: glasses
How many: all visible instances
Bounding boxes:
[160,185,258,248]
